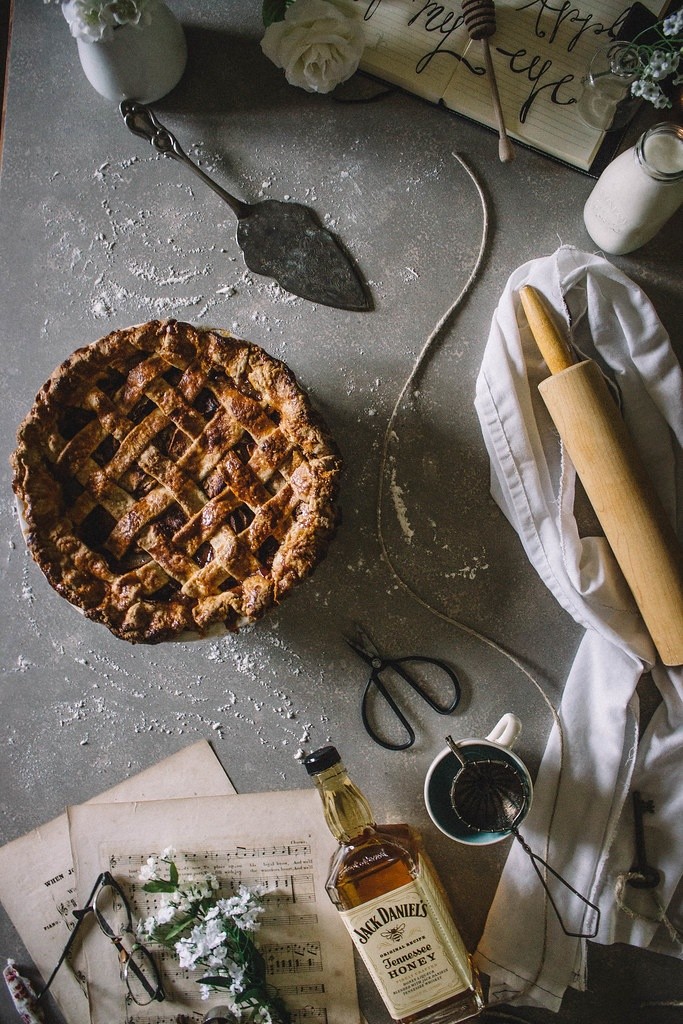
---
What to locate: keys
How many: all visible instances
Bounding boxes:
[628,790,662,891]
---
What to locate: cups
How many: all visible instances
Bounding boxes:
[424,713,534,846]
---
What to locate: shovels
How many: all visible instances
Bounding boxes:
[119,100,372,313]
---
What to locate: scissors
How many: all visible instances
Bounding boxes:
[340,621,462,751]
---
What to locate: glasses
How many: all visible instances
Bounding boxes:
[29,872,166,1013]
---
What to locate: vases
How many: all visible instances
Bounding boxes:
[77,0,189,105]
[576,40,645,131]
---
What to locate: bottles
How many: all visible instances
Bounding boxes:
[576,40,650,133]
[583,120,683,255]
[303,745,485,1024]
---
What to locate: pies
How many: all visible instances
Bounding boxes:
[10,318,344,647]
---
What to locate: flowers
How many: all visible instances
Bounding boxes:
[260,0,365,93]
[593,8,683,109]
[135,845,293,1024]
[44,0,161,45]
